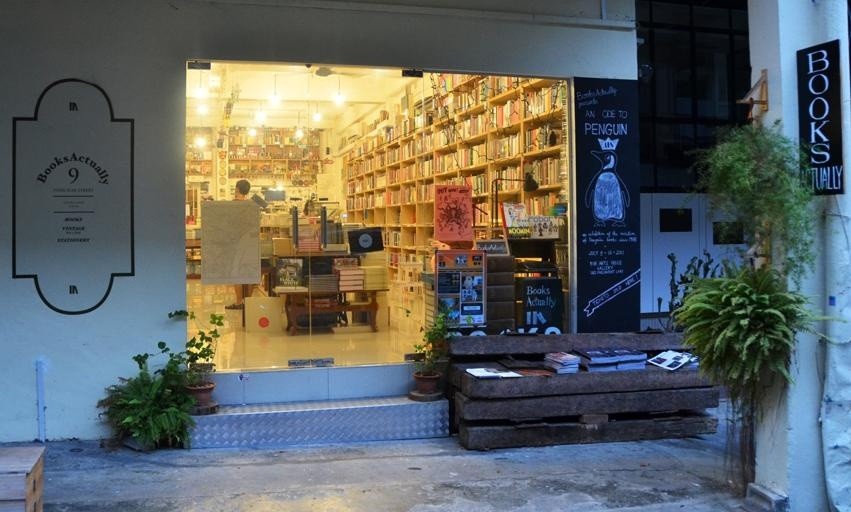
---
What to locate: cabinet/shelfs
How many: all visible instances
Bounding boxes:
[0,446,46,512]
[186,127,213,177]
[229,127,324,180]
[341,73,568,333]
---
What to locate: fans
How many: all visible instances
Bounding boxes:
[279,66,366,77]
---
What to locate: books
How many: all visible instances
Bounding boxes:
[271,217,390,292]
[447,74,566,238]
[345,92,447,210]
[466,348,699,381]
[383,232,429,282]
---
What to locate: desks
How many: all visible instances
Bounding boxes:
[241,251,367,327]
[273,286,390,336]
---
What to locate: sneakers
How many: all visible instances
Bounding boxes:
[225,302,245,309]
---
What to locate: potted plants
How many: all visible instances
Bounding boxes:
[168,310,224,415]
[413,312,450,394]
[96,353,197,451]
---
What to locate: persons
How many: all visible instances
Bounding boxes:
[303,200,316,217]
[225,180,255,311]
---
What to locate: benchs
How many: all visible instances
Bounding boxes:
[447,333,720,450]
